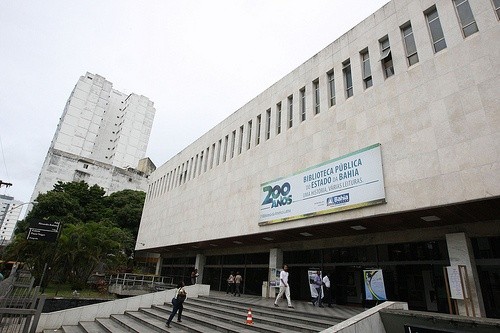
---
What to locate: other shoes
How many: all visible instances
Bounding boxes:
[165,325,171,328]
[177,319,182,322]
[288,306,294,308]
[312,300,315,305]
[319,305,324,308]
[274,303,279,307]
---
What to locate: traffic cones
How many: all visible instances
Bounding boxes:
[244,306,255,324]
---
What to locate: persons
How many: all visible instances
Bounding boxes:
[274,265,294,309]
[323,273,334,308]
[226,272,234,295]
[312,271,324,307]
[166,281,187,328]
[191,269,198,285]
[233,272,242,297]
